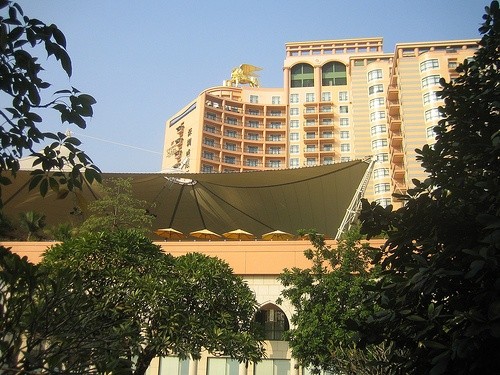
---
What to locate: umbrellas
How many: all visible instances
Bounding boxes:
[154,228,323,242]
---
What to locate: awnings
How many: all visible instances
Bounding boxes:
[1,157,377,249]
